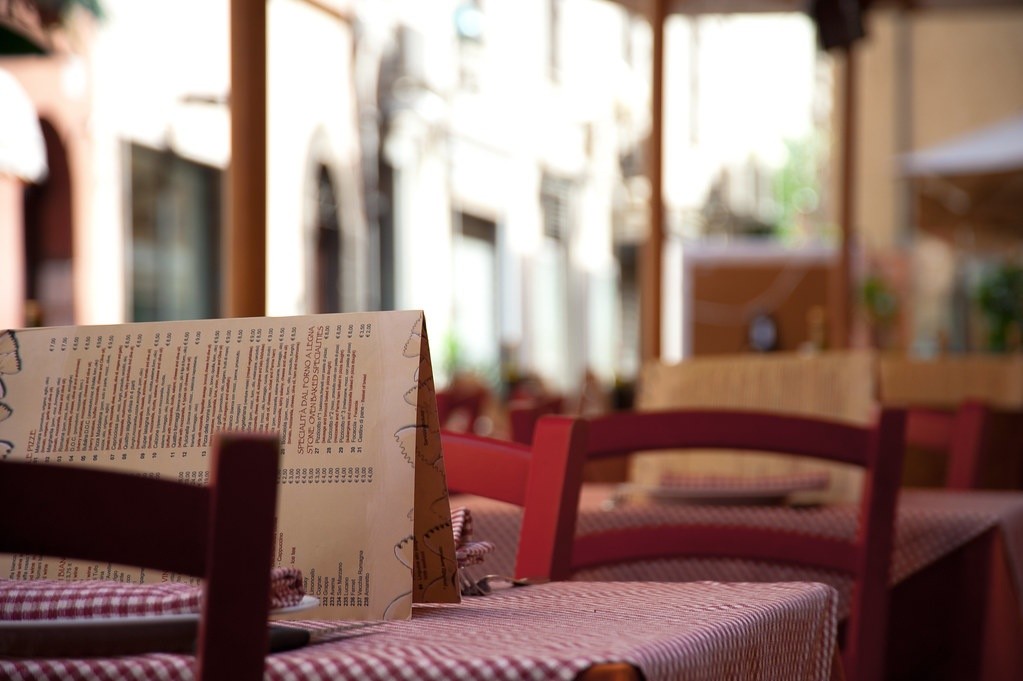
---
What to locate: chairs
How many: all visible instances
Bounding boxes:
[0,390,1004,681]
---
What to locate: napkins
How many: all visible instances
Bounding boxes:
[651,468,826,489]
[1,566,307,621]
[451,508,495,568]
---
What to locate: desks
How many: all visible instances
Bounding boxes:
[0,578,853,681]
[445,482,1023,681]
